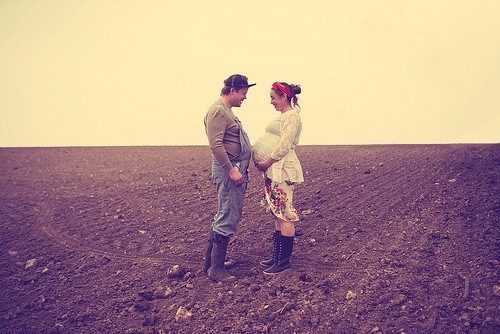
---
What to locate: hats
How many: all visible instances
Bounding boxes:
[225,74,256,87]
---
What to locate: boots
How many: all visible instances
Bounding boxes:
[263,233,294,274]
[207,233,237,282]
[202,227,233,272]
[260,230,281,267]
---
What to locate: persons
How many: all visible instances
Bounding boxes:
[251,81,304,275]
[201,74,256,283]
